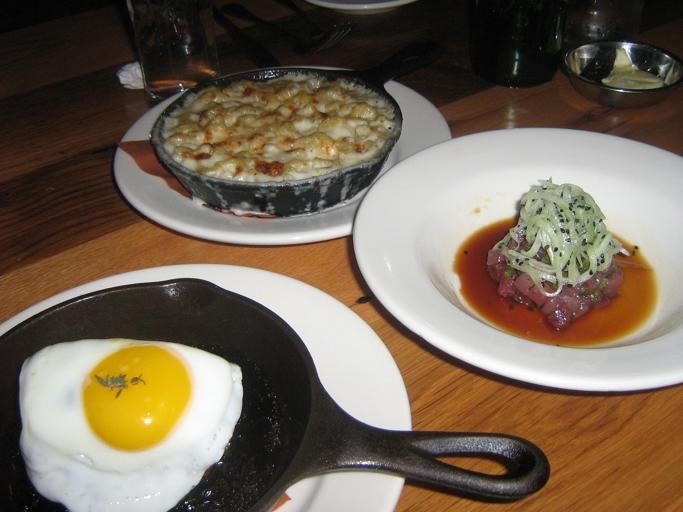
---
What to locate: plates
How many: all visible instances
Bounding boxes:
[350,124,683,396]
[0,263,414,512]
[109,63,451,249]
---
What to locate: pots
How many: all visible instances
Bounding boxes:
[148,38,451,221]
[0,276,552,512]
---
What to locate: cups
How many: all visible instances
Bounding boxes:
[125,0,225,105]
[467,1,569,90]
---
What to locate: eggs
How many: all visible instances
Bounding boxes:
[18,339,243,511]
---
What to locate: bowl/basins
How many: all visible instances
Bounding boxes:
[562,35,683,110]
